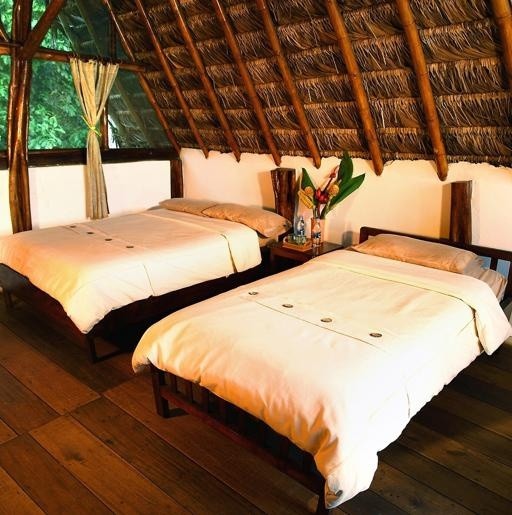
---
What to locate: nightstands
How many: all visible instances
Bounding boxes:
[265,239,344,271]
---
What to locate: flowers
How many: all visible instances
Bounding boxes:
[297,147,369,217]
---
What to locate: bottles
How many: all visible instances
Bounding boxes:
[311,219,321,248]
[296,216,305,235]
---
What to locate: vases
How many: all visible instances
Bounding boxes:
[308,217,326,246]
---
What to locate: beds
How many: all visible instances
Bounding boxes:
[124,224,511,508]
[0,198,289,370]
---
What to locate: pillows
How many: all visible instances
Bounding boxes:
[159,197,217,217]
[352,230,481,278]
[203,202,292,237]
